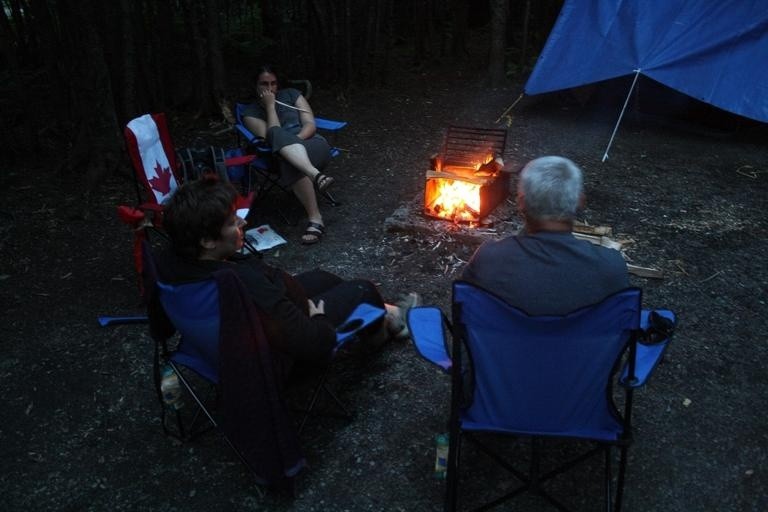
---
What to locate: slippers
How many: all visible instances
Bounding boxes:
[315,174,334,190]
[302,221,325,243]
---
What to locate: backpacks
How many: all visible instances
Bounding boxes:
[177,144,230,187]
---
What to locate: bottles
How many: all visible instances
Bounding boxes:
[433,424,450,480]
[160,365,184,437]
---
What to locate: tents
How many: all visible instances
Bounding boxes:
[240,65,334,242]
[495,0,768,163]
[177,185,423,355]
[462,155,631,314]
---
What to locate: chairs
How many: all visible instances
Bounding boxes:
[136,218,387,506]
[235,99,350,208]
[116,112,256,297]
[407,278,676,512]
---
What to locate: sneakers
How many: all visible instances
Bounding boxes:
[354,332,387,355]
[388,292,422,340]
